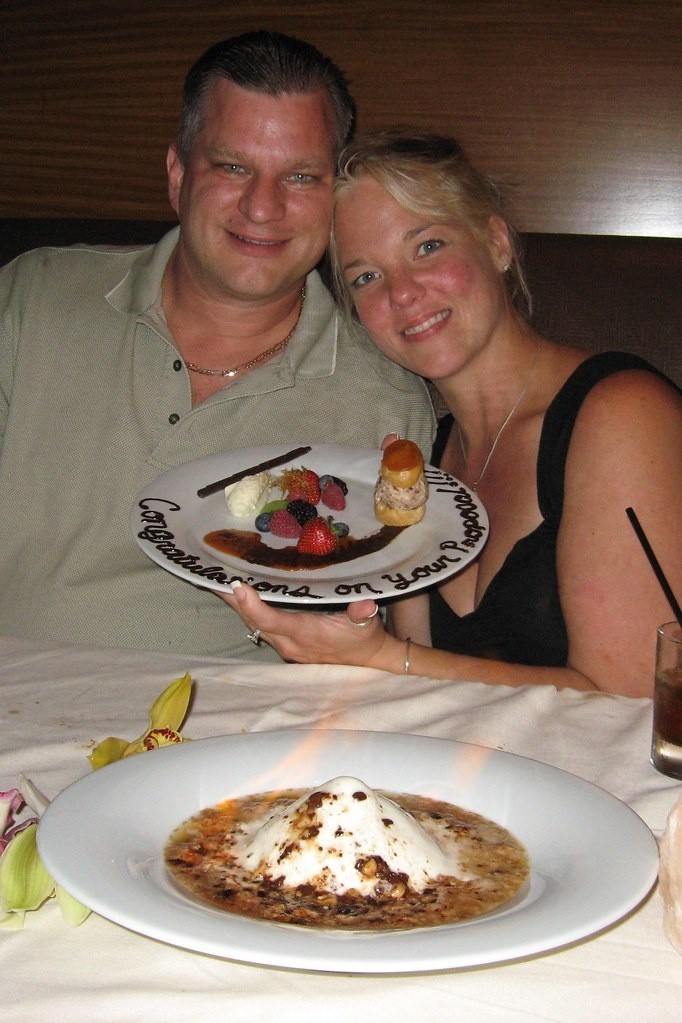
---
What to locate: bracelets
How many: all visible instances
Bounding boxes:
[405,637,411,675]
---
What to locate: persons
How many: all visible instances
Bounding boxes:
[211,123,682,699]
[0,29,435,665]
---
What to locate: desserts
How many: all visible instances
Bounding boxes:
[373,439,429,526]
[164,775,527,929]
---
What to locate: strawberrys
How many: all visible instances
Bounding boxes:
[267,469,345,555]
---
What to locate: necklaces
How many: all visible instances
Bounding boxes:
[456,333,541,495]
[184,285,305,376]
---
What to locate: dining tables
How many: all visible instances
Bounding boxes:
[0,639,682,1023]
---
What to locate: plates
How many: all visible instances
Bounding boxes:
[35,729,661,975]
[129,442,490,605]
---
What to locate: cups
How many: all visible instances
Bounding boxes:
[649,622,682,781]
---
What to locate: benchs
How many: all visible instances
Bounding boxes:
[0,220,682,413]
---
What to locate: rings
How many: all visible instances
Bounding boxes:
[349,617,374,628]
[246,630,260,644]
